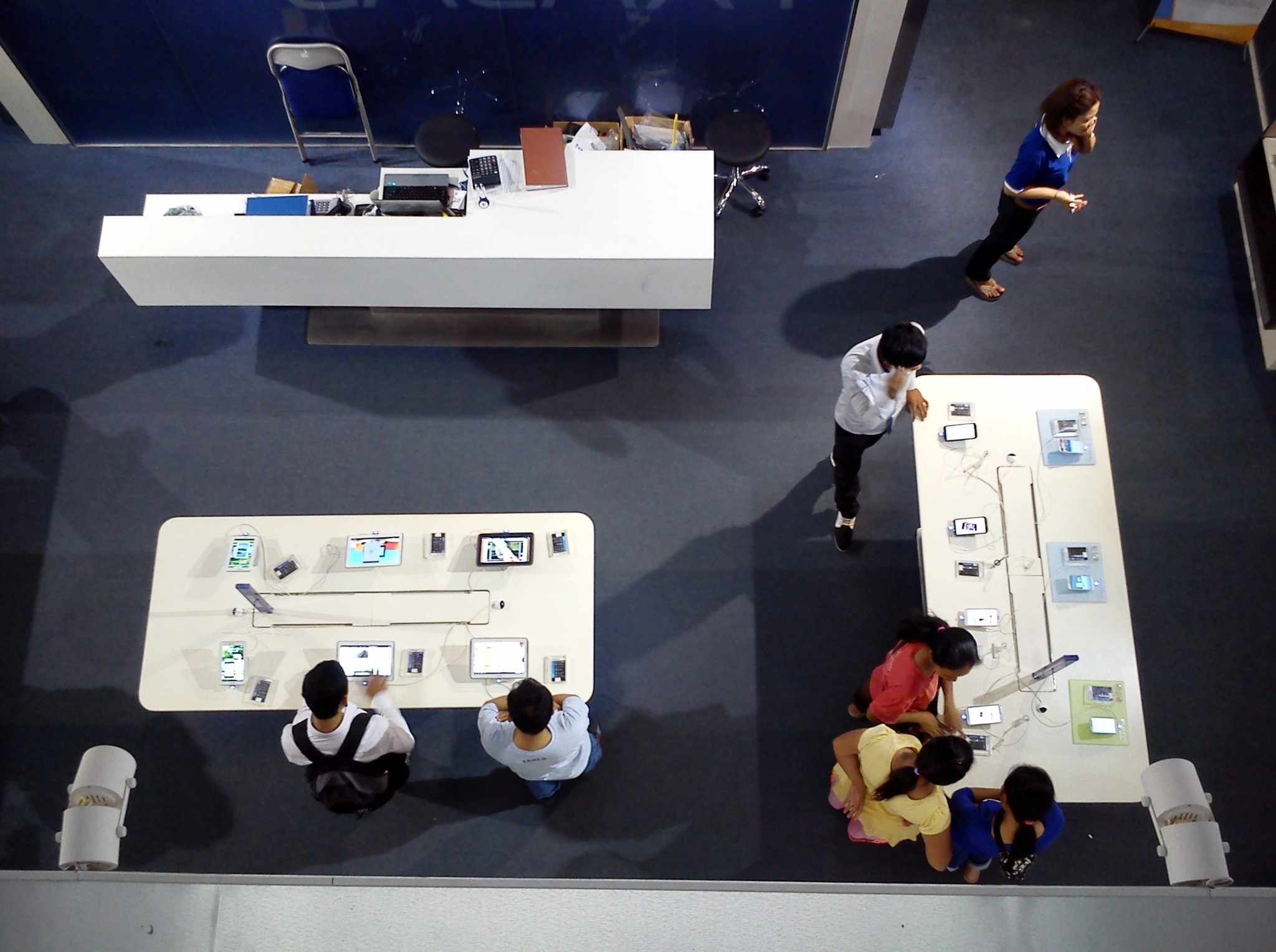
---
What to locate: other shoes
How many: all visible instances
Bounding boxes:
[848,703,865,718]
[946,861,960,871]
[595,733,605,746]
[849,834,889,844]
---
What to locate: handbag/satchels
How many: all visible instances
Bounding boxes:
[992,850,1038,883]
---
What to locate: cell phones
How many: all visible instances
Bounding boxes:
[1089,685,1113,702]
[965,704,1004,726]
[962,734,988,751]
[964,608,998,627]
[956,562,982,578]
[1064,547,1088,562]
[1057,439,1083,454]
[1067,574,1095,591]
[953,516,988,536]
[944,423,977,442]
[1089,717,1119,735]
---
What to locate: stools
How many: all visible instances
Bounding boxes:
[704,111,771,219]
[414,113,480,169]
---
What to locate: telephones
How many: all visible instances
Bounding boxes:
[314,197,352,216]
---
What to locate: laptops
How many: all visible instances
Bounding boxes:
[371,173,449,213]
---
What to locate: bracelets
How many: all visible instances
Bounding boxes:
[1055,190,1060,199]
[1089,133,1095,140]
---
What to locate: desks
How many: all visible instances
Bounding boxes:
[97,149,714,346]
[909,375,1149,804]
[138,513,594,712]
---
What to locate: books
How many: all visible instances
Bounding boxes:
[519,127,568,191]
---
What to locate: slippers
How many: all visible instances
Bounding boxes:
[965,274,1004,299]
[1001,246,1024,265]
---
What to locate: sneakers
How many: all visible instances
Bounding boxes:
[830,443,837,466]
[834,510,857,551]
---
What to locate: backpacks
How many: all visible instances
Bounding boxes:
[291,713,410,815]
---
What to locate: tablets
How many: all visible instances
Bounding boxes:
[345,533,403,568]
[476,533,534,567]
[219,642,248,685]
[336,641,395,681]
[469,638,528,678]
[224,536,258,571]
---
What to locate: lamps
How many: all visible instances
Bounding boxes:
[1141,757,1234,887]
[55,744,136,871]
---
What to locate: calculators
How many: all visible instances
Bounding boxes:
[470,155,500,189]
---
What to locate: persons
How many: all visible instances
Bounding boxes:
[849,607,981,738]
[828,724,973,871]
[281,660,415,767]
[964,78,1101,301]
[830,322,928,552]
[478,678,602,799]
[947,765,1065,883]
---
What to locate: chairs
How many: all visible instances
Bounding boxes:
[267,43,381,165]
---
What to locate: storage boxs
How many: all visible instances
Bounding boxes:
[544,121,620,150]
[620,116,693,151]
